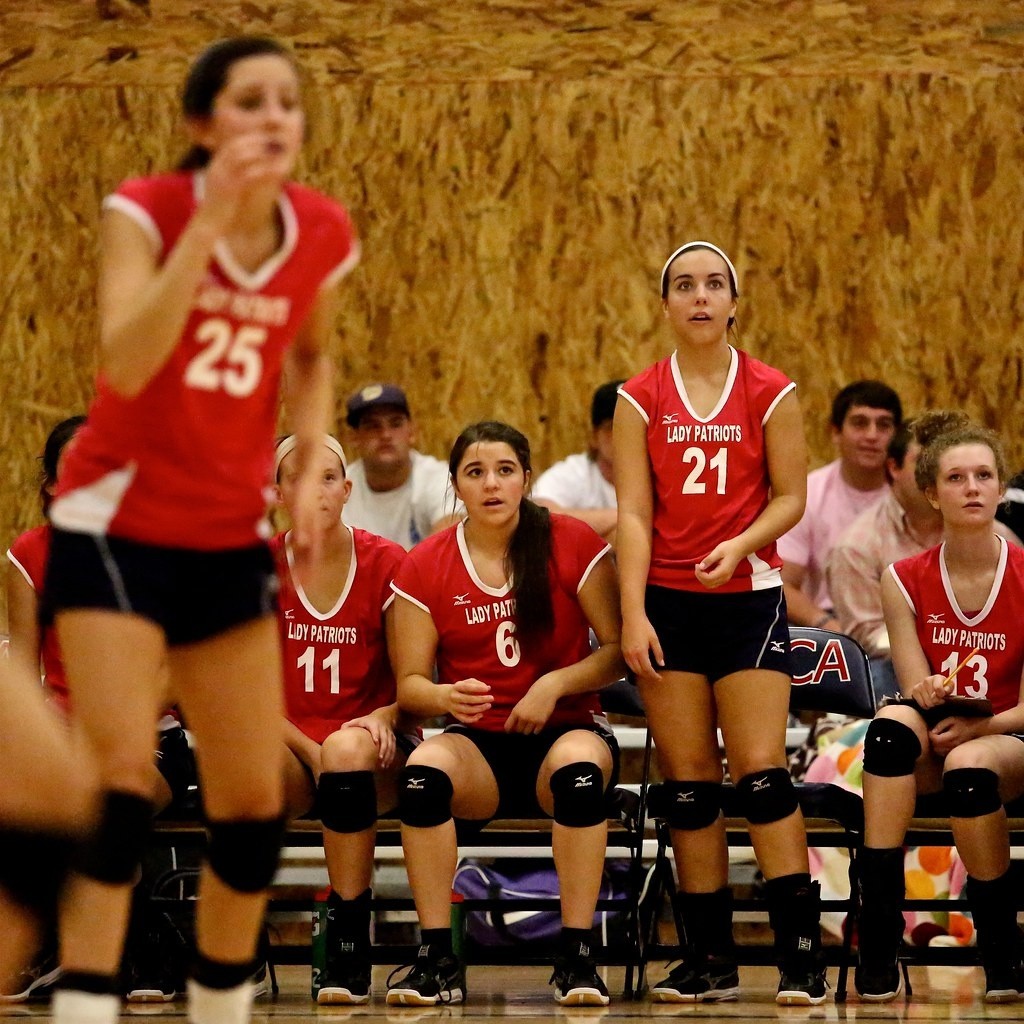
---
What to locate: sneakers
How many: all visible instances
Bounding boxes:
[122,956,185,1005]
[385,945,467,1005]
[549,940,610,1005]
[0,940,63,1003]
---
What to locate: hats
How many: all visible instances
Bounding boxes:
[346,385,409,429]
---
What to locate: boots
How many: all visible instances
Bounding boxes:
[315,888,374,1004]
[762,881,831,1004]
[967,864,1024,1003]
[651,887,741,1002]
[855,844,907,1004]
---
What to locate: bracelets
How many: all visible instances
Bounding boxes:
[815,613,836,628]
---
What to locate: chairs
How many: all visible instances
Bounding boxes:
[154,627,1023,997]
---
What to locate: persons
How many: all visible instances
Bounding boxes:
[528,378,631,557]
[385,422,628,1005]
[613,243,828,1007]
[1,413,191,1002]
[46,32,361,1024]
[252,434,422,1003]
[0,628,99,1002]
[831,419,1024,710]
[341,385,467,552]
[777,381,903,633]
[846,409,1024,1004]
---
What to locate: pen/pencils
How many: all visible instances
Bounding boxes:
[931,647,980,698]
